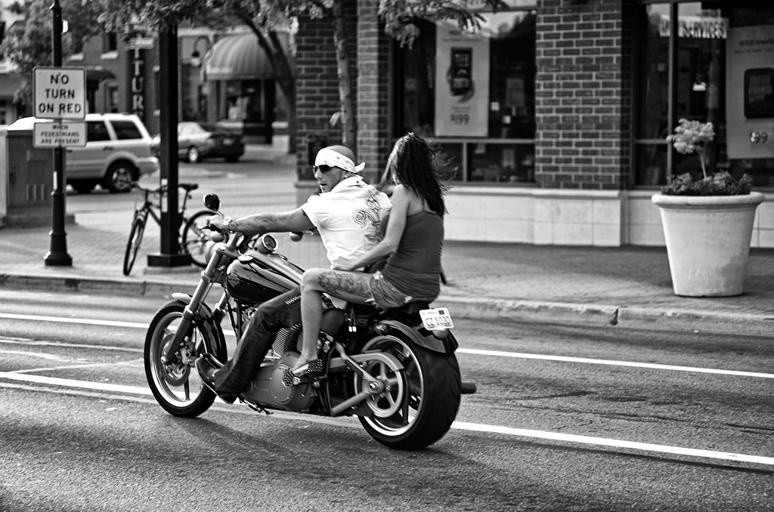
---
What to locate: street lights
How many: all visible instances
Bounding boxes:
[190,36,210,67]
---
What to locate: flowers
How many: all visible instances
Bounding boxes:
[662,113,757,196]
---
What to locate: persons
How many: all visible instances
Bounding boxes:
[281,131,456,388]
[195,145,392,404]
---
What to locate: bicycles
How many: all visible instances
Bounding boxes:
[119,179,230,276]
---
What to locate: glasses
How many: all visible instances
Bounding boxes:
[311,164,336,173]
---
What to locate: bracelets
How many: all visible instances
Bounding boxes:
[226,217,236,234]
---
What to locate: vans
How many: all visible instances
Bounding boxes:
[6,112,160,194]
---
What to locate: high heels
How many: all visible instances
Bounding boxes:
[279,357,325,397]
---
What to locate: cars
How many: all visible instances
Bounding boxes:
[153,122,245,163]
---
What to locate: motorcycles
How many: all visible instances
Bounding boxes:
[143,224,477,450]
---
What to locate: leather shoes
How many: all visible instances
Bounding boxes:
[194,356,235,405]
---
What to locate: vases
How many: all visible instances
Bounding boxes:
[651,193,764,298]
